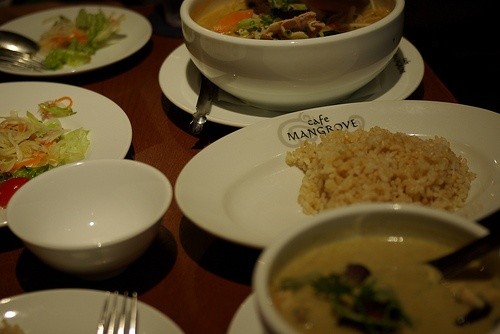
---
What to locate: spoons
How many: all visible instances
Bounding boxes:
[0,30,40,54]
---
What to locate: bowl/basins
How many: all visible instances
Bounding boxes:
[180,0,405,111]
[252,202,500,334]
[7,159,173,280]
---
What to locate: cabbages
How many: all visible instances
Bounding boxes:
[0,95,91,179]
[28,7,126,68]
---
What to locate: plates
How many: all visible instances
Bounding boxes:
[0,288,185,334]
[158,37,426,128]
[0,82,132,228]
[227,294,264,334]
[174,100,500,250]
[0,5,153,77]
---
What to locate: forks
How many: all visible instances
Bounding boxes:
[96,290,137,334]
[0,48,44,72]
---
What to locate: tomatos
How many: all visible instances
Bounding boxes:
[0,177,30,207]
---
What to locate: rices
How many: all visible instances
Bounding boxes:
[285,126,477,215]
